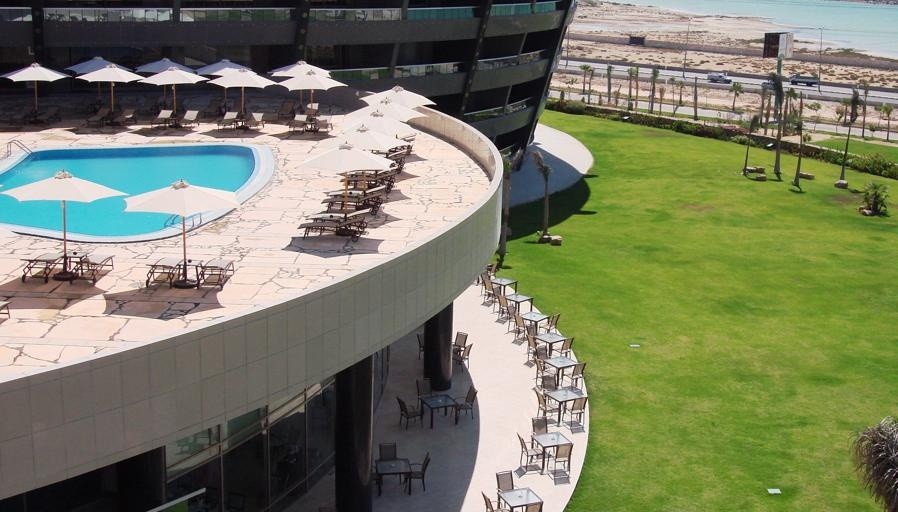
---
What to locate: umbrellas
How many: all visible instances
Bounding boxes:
[0,169,130,272]
[123,179,240,279]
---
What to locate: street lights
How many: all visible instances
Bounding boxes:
[683,16,695,77]
[818,26,826,93]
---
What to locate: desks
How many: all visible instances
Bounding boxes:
[418,394,459,429]
[62,252,89,276]
[375,458,412,496]
[176,260,204,282]
[453,344,461,365]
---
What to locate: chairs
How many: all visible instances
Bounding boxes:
[146,257,184,288]
[371,466,384,488]
[453,332,468,356]
[198,257,235,291]
[0,299,14,318]
[379,441,397,461]
[477,263,588,512]
[453,343,473,373]
[69,253,115,285]
[297,137,415,242]
[397,396,424,430]
[416,333,425,360]
[416,378,432,409]
[403,451,431,493]
[20,253,64,284]
[450,385,478,420]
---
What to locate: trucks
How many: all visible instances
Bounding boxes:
[707,71,732,84]
[789,74,819,86]
